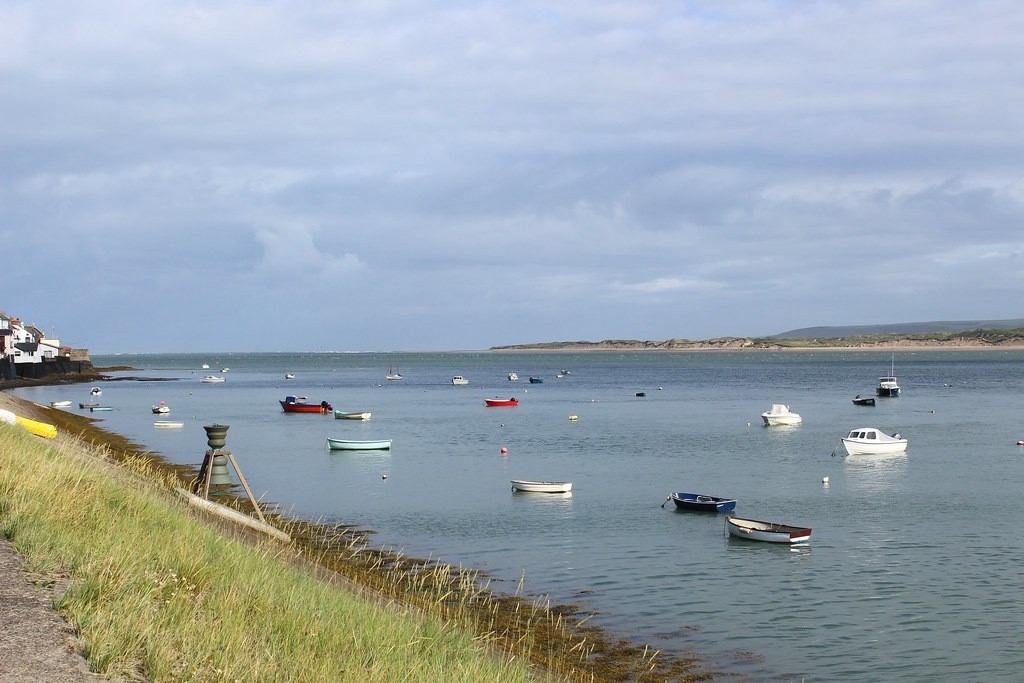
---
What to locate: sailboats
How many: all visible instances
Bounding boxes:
[385,359,403,380]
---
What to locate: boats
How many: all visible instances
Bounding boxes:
[510,479,572,493]
[220,367,230,373]
[199,374,226,383]
[202,362,209,369]
[452,375,469,385]
[556,373,563,378]
[670,491,738,513]
[327,437,392,451]
[285,373,295,379]
[90,406,113,412]
[51,399,73,407]
[725,515,813,543]
[568,415,577,421]
[875,352,901,397]
[78,403,100,409]
[636,392,647,397]
[153,420,184,429]
[333,409,372,420]
[484,397,518,407]
[761,403,802,426]
[529,377,544,384]
[90,386,102,396]
[507,372,519,381]
[278,395,325,413]
[840,428,908,456]
[561,369,571,375]
[152,405,170,413]
[852,394,878,407]
